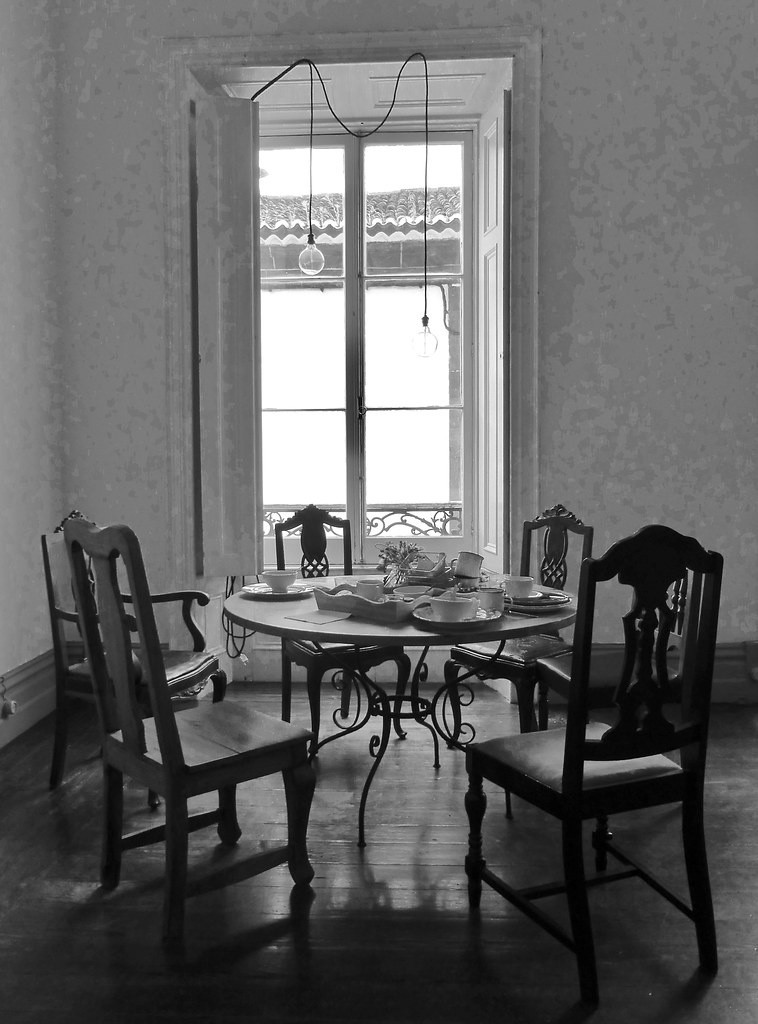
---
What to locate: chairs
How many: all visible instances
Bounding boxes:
[276,504,406,726]
[443,505,600,761]
[41,518,235,813]
[463,524,725,1003]
[55,520,317,953]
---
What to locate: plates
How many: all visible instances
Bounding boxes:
[504,591,572,612]
[240,581,314,599]
[412,607,502,628]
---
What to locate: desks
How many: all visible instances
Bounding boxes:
[221,574,582,850]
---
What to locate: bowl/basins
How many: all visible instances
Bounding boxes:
[392,585,434,600]
[261,570,298,593]
[457,578,479,588]
[504,576,534,598]
[431,596,473,622]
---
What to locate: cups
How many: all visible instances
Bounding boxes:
[356,579,385,602]
[450,551,484,578]
[478,587,513,613]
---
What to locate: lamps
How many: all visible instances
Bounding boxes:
[412,56,439,359]
[297,61,327,278]
